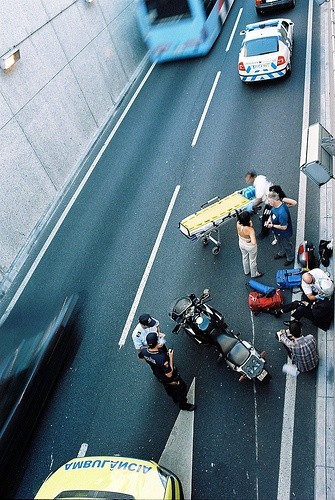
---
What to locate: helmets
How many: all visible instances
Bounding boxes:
[315,278,334,297]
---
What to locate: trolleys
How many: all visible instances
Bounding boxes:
[178,185,262,256]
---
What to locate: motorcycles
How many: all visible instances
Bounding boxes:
[166,287,273,385]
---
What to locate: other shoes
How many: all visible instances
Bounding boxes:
[256,271,264,278]
[272,239,278,245]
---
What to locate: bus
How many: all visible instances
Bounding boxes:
[133,0,235,62]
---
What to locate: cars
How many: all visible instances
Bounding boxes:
[238,17,295,84]
[255,0,296,14]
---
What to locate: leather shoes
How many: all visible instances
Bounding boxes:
[285,261,292,265]
[283,320,296,326]
[274,254,281,259]
[271,309,282,317]
[180,403,196,411]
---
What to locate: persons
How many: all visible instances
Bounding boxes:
[244,169,274,240]
[138,332,196,411]
[264,191,295,267]
[131,313,178,383]
[269,185,297,246]
[237,211,265,278]
[272,268,335,333]
[280,320,320,375]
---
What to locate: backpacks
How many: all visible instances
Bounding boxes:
[249,288,283,311]
[276,269,302,288]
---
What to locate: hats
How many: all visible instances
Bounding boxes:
[146,333,157,348]
[139,313,156,327]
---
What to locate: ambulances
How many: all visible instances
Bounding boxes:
[31,457,184,500]
